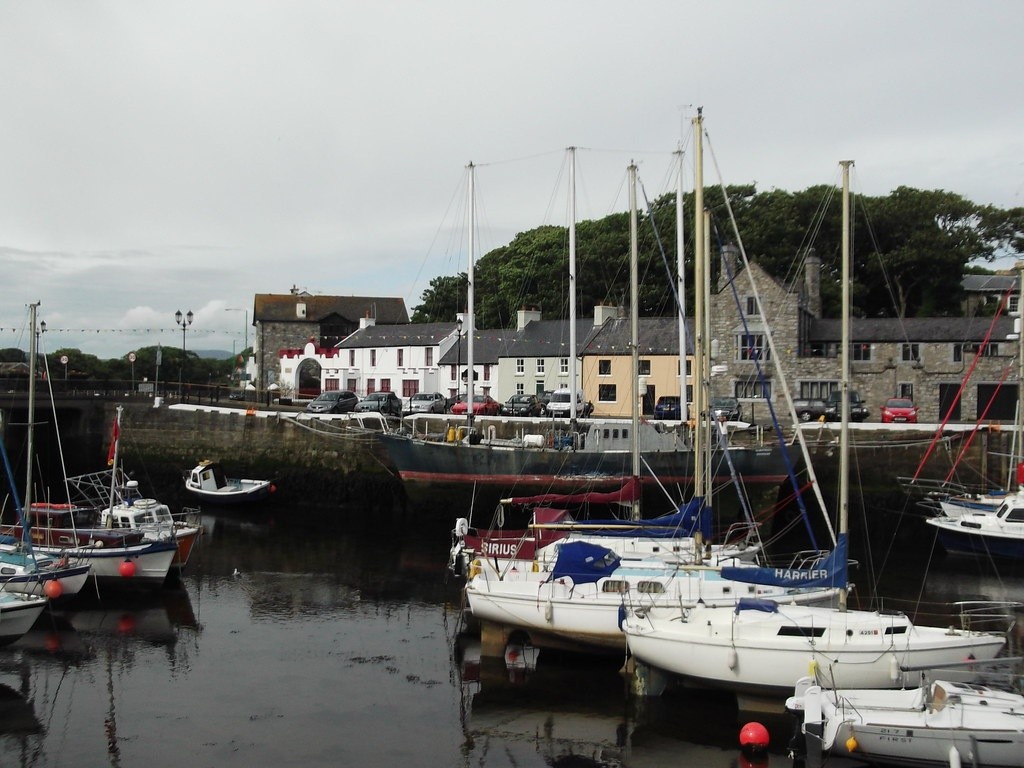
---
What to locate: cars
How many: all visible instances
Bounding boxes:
[789,396,826,424]
[401,392,448,415]
[700,396,746,422]
[451,394,503,416]
[306,390,358,414]
[879,398,920,423]
[229,389,244,400]
[501,394,544,417]
[652,395,691,421]
[446,393,468,410]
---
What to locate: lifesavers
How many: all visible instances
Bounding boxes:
[455,518,469,537]
[470,560,481,579]
[32,502,76,509]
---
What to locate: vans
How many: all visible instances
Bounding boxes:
[545,387,585,419]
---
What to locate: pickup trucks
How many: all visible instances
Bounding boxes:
[352,390,402,418]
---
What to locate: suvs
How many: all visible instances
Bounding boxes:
[821,389,872,422]
[537,389,594,419]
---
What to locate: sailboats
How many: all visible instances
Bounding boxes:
[896,267,1023,540]
[1,299,205,652]
[448,103,1008,692]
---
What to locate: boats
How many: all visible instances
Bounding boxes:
[184,458,274,515]
[785,656,1024,768]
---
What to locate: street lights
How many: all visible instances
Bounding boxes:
[174,308,193,402]
[30,319,47,377]
